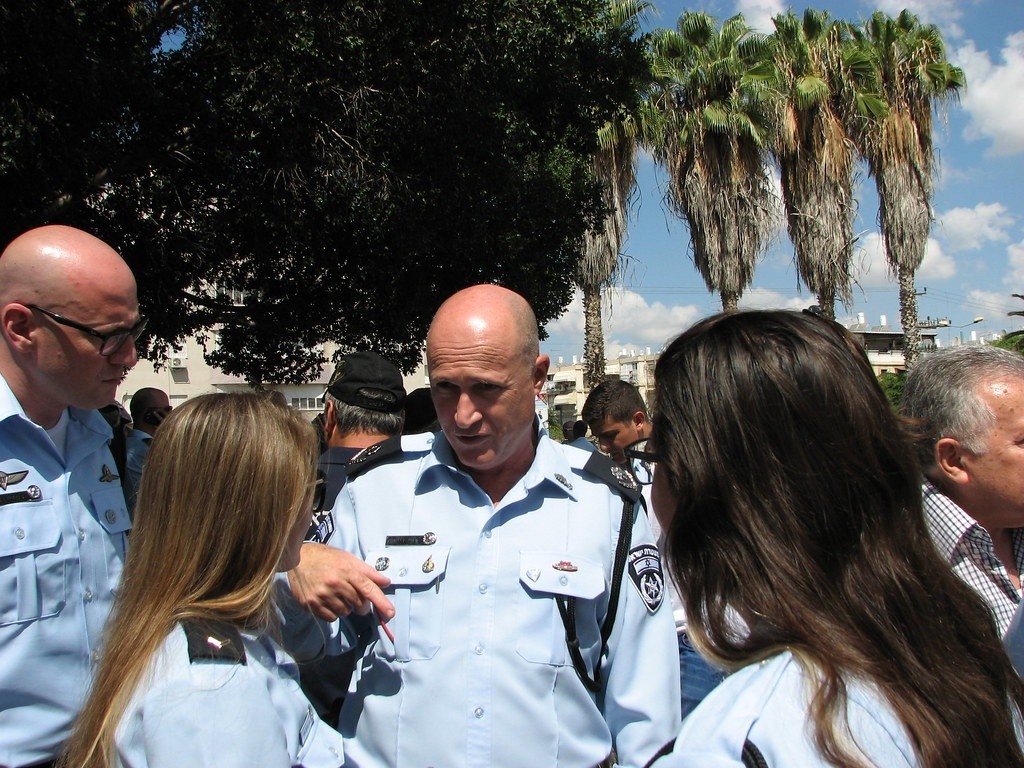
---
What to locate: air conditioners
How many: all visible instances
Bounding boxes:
[171,357,187,368]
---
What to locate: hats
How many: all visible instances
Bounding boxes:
[322,350,408,415]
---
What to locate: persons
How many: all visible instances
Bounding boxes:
[561,420,598,452]
[624,309,1024,768]
[897,342,1024,642]
[118,389,173,520]
[265,284,679,768]
[581,380,723,720]
[303,353,407,543]
[0,226,138,768]
[63,392,345,768]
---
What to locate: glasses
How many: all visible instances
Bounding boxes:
[622,437,670,486]
[563,427,573,432]
[24,303,151,358]
[149,406,172,413]
